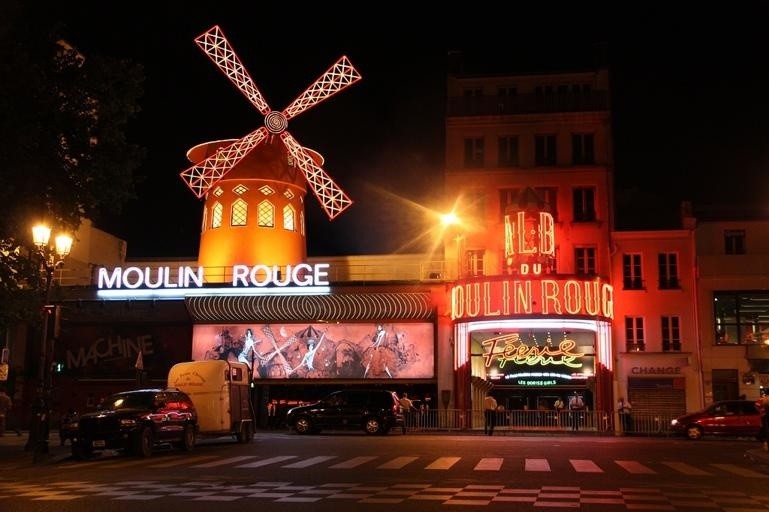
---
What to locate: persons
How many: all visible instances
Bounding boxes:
[539,398,549,426]
[0,389,13,437]
[618,397,631,432]
[400,392,419,434]
[391,333,406,361]
[265,401,275,431]
[288,331,325,376]
[238,328,265,370]
[420,398,431,427]
[484,392,515,435]
[753,388,768,452]
[363,324,393,378]
[553,396,565,427]
[570,392,583,430]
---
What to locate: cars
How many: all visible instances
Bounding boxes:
[669,400,766,443]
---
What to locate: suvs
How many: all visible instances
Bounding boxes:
[286,390,404,435]
[65,387,201,459]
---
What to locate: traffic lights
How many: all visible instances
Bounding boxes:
[49,359,67,376]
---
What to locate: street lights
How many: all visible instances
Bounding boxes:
[26,217,74,457]
